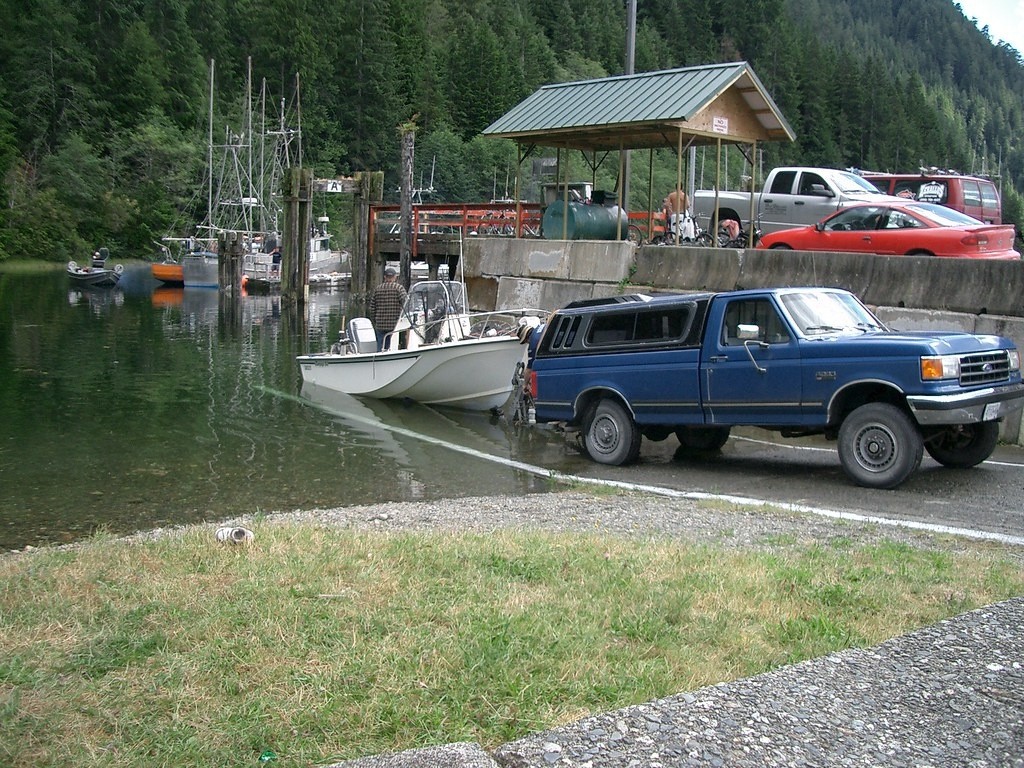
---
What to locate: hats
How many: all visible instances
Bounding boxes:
[384,268,396,276]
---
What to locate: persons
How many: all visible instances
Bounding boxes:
[665,182,689,213]
[369,268,408,352]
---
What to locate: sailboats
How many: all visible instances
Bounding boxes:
[153,55,352,289]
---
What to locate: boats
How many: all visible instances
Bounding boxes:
[296,279,527,412]
[67,248,124,290]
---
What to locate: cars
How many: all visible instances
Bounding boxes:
[752,197,1020,262]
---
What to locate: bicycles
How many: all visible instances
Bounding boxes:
[627,212,764,249]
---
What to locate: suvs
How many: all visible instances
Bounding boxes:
[525,286,1024,487]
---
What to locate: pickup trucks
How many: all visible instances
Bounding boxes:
[694,165,891,239]
